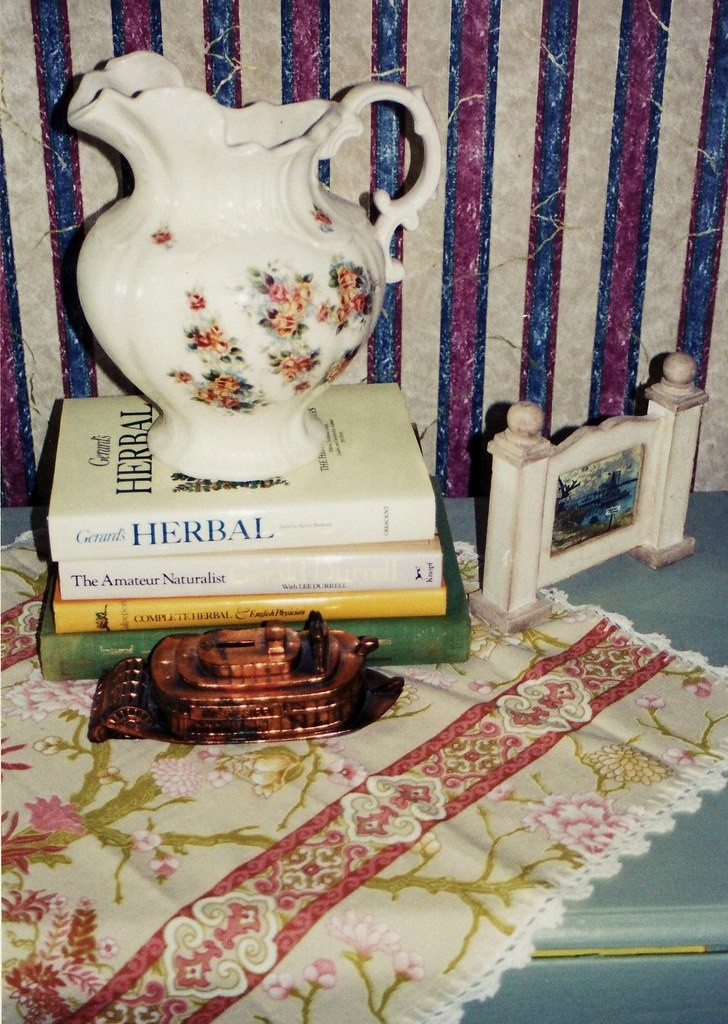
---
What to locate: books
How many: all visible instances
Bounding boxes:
[30,381,473,682]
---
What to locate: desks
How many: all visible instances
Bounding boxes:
[2,492,728,1024]
